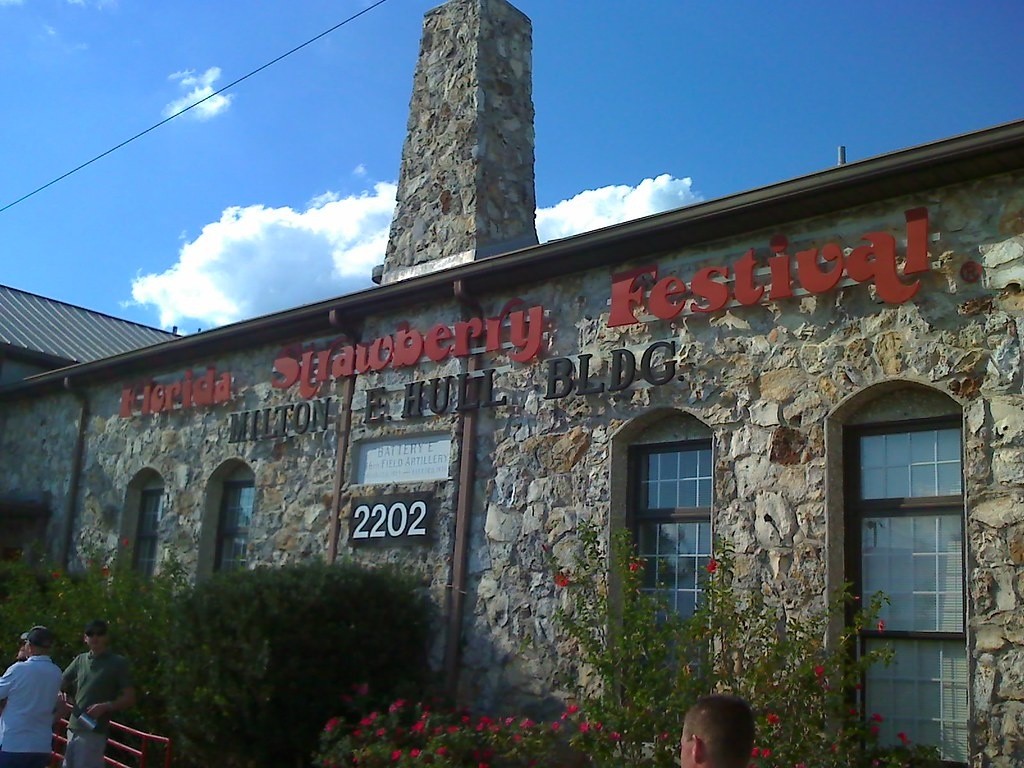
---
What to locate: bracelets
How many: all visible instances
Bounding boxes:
[107,701,113,711]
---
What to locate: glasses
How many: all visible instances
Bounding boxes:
[85,630,106,637]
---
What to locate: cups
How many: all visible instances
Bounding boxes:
[76,713,96,730]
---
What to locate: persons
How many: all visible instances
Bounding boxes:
[681,695,755,768]
[60,619,135,768]
[0,624,62,767]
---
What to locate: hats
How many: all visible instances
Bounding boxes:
[20,626,58,648]
[85,619,108,633]
